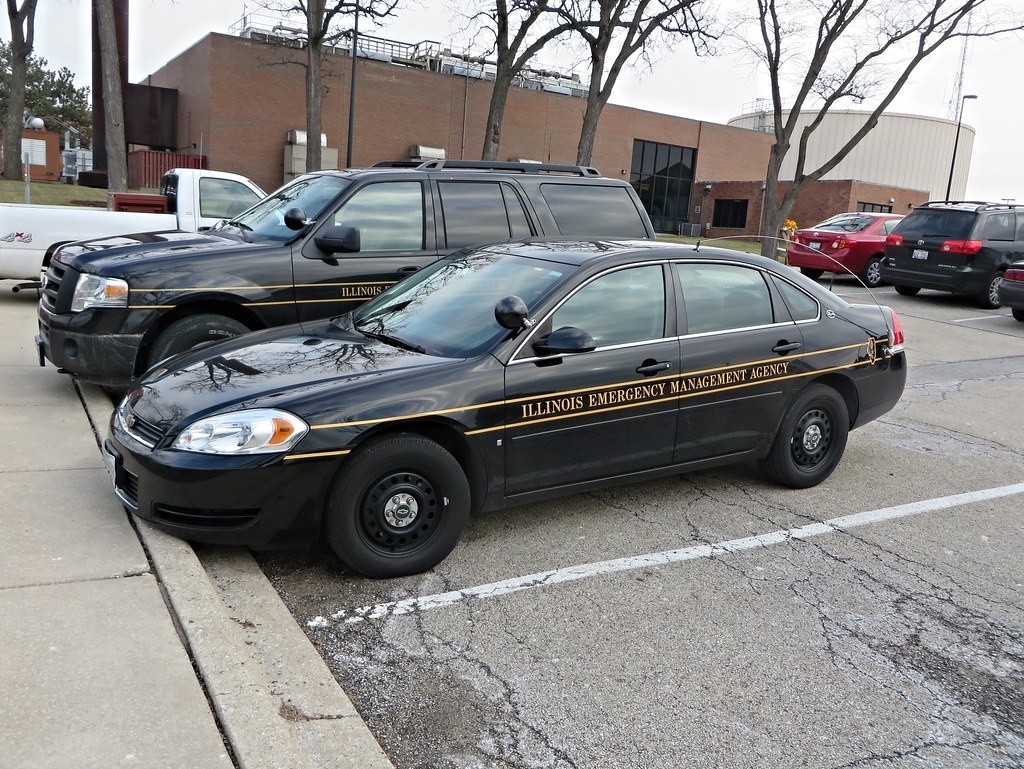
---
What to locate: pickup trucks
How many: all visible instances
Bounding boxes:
[0,168,343,293]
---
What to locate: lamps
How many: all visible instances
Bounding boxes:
[705,182,712,192]
[890,197,895,203]
[621,169,628,174]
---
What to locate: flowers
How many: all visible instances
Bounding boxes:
[780,219,798,239]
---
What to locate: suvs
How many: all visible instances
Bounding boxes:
[879,198,1024,311]
[33,157,659,389]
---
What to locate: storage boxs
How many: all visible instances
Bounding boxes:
[107,192,168,213]
[681,223,701,236]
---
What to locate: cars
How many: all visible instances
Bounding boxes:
[102,236,909,579]
[787,212,908,288]
[997,260,1024,323]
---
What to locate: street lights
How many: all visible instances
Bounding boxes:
[1000,198,1015,204]
[944,94,978,205]
